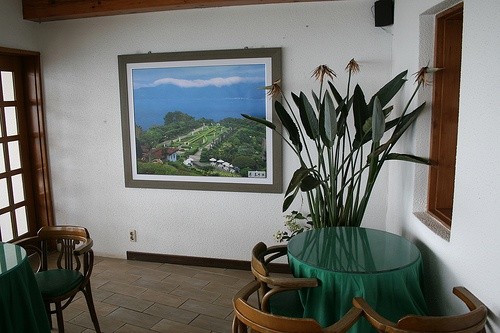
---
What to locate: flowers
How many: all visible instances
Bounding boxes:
[273,211,304,241]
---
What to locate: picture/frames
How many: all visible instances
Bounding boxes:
[118,47,283,193]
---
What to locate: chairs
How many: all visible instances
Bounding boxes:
[12,225,104,333]
[230,279,361,333]
[353,287,487,333]
[251,242,319,317]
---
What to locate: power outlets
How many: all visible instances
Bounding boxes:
[129,230,136,243]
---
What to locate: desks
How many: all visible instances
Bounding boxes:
[286,226,427,333]
[0,242,52,333]
